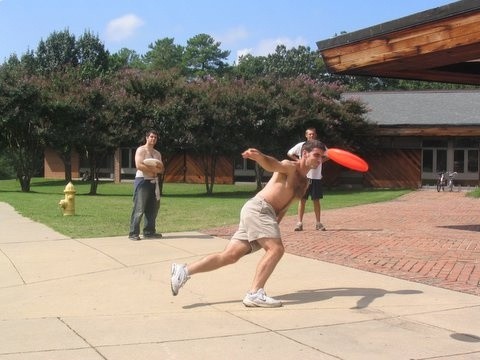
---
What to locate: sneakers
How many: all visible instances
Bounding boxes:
[170,262,191,295]
[295,222,303,231]
[316,224,325,231]
[144,233,162,239]
[243,288,282,308]
[129,235,140,241]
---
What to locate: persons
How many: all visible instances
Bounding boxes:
[271,259,275,261]
[127,131,164,241]
[168,138,327,309]
[286,126,329,232]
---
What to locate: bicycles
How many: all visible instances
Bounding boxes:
[446,171,457,192]
[436,172,445,192]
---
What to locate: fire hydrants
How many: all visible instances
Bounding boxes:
[59,181,77,216]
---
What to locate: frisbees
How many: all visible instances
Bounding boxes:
[327,148,369,172]
